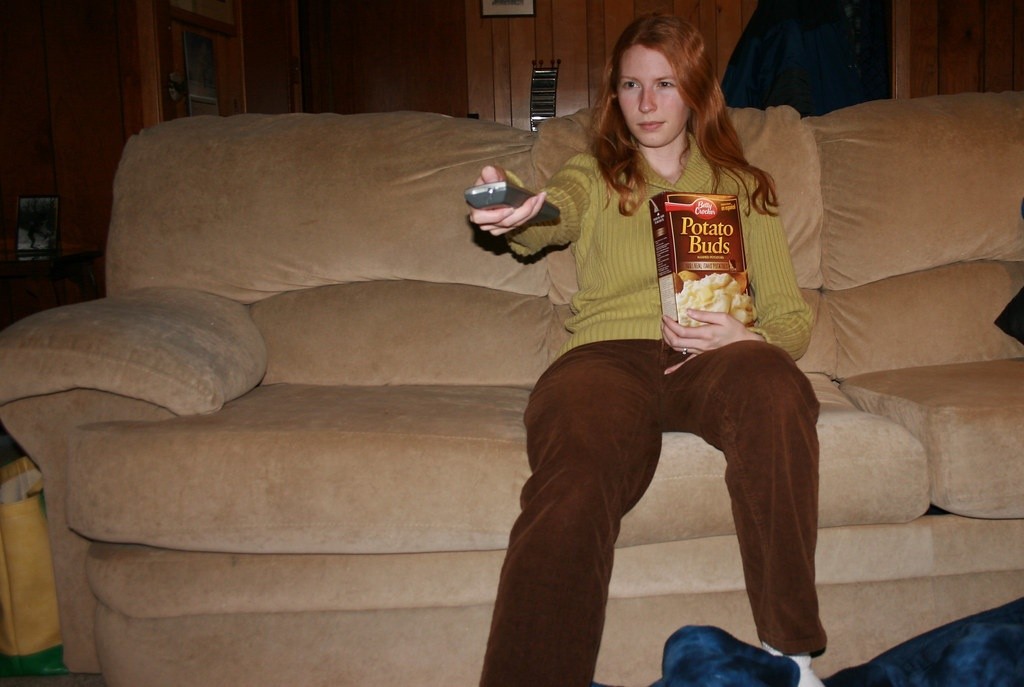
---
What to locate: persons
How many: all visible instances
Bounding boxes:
[721,0,893,119]
[28,221,36,247]
[470,12,827,687]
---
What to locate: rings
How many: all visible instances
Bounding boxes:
[683,348,687,355]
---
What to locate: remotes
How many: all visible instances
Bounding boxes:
[465,180,561,223]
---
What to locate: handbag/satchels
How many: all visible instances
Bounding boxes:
[0,455,68,675]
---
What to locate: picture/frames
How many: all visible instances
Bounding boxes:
[14,193,62,260]
[479,0,537,19]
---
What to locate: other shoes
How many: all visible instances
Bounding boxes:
[762,641,825,687]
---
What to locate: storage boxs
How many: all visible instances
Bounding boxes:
[647,193,756,328]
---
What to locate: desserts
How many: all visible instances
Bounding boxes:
[673,270,757,328]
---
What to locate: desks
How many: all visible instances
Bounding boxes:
[0,245,103,331]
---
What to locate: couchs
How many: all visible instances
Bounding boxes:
[2,91,1023,686]
